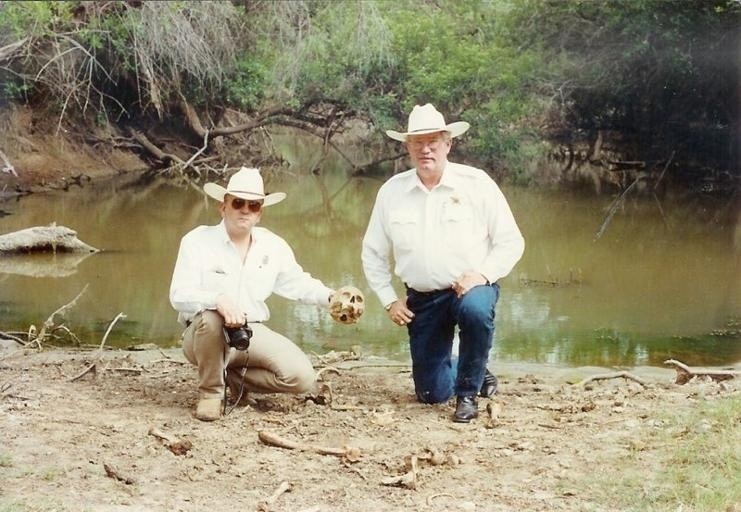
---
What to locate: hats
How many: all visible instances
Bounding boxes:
[203,166,287,208]
[385,102,471,143]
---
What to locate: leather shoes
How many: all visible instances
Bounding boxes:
[452,393,479,423]
[480,369,498,398]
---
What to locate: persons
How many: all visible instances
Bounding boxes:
[358,103,525,420]
[168,164,363,422]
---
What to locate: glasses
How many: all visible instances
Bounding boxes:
[232,199,260,212]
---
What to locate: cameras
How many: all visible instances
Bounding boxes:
[223,314,253,350]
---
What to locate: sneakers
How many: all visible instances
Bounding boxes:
[225,375,258,407]
[195,398,222,421]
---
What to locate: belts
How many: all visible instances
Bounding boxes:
[404,282,453,297]
